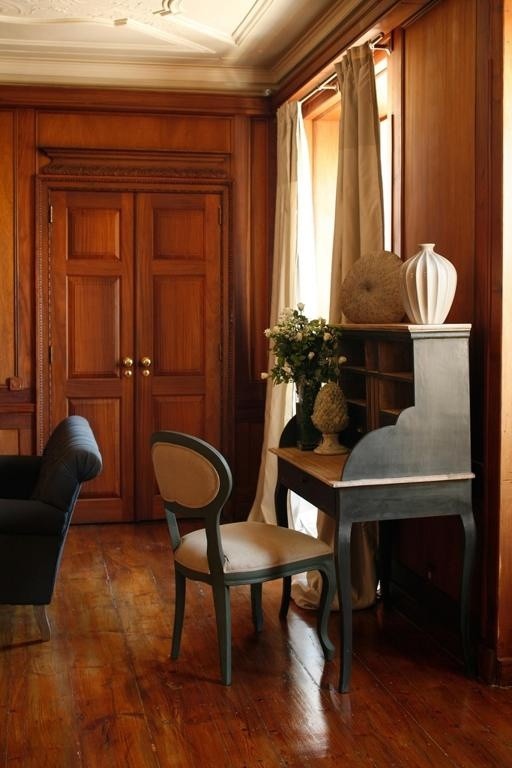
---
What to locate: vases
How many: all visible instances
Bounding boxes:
[260,302,348,406]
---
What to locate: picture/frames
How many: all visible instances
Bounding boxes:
[259,321,478,693]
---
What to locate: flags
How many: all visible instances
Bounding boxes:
[294,394,323,451]
[397,242,459,326]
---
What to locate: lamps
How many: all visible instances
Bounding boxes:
[1,413,103,644]
[149,429,339,687]
